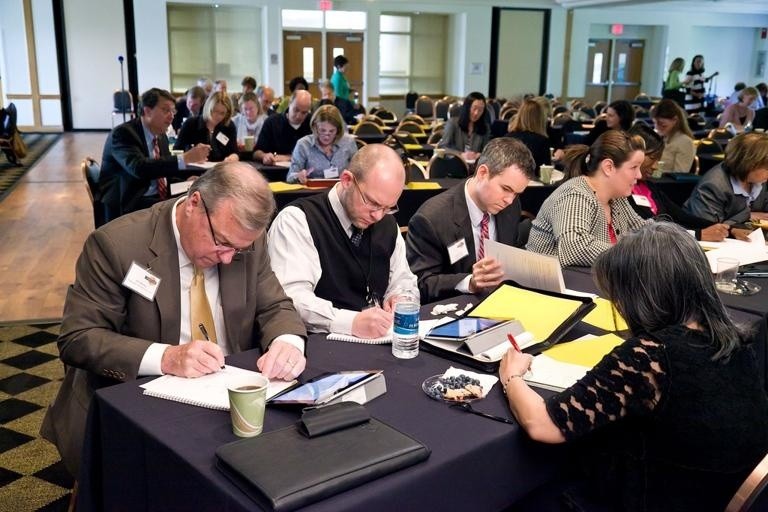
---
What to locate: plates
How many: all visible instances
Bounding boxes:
[421,373,484,403]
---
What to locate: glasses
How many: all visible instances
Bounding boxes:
[200,197,255,256]
[352,174,400,215]
[147,99,340,138]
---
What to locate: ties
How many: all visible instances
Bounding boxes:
[189,267,218,343]
[351,227,366,247]
[479,213,491,264]
[152,138,168,201]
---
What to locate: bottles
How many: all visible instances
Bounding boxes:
[390,273,422,358]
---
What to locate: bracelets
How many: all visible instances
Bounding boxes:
[503,375,518,394]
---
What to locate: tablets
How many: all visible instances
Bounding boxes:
[426,316,515,341]
[267,370,381,403]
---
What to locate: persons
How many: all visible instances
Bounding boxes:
[40,158,309,510]
[524,130,653,268]
[404,137,536,306]
[663,55,705,117]
[498,220,768,511]
[331,55,356,99]
[96,75,768,240]
[265,143,419,337]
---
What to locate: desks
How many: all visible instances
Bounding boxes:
[568,247,766,319]
[80,269,767,510]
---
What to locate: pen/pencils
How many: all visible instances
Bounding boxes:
[198,323,226,370]
[714,212,720,223]
[507,335,534,377]
[306,168,315,175]
[274,152,276,166]
[190,144,212,151]
[372,291,380,309]
[478,258,486,271]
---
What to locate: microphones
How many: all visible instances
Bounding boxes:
[708,71,718,81]
[118,56,126,63]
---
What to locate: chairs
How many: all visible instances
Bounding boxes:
[0,102,19,166]
[720,453,768,509]
[80,91,768,241]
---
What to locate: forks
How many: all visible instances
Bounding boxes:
[447,402,512,427]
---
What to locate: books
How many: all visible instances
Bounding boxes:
[326,319,439,345]
[144,364,298,412]
[215,411,430,512]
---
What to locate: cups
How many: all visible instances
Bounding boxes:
[572,111,585,121]
[653,161,666,179]
[538,164,553,184]
[228,374,270,438]
[715,257,739,290]
[243,135,255,152]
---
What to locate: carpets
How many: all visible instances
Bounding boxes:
[0,130,66,204]
[1,318,87,510]
